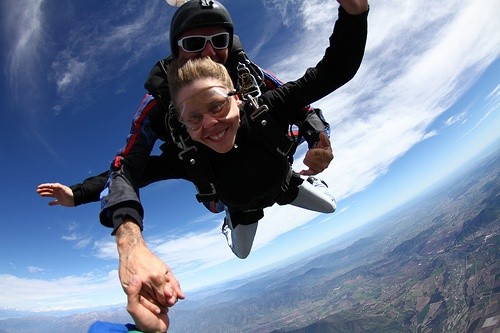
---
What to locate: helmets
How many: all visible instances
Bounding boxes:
[170,0,234,57]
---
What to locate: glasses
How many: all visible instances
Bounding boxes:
[177,85,240,133]
[178,32,230,52]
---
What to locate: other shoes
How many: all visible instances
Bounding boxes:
[307,177,328,187]
[222,217,226,232]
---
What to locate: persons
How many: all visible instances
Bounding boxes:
[36,0,371,259]
[99,0,334,333]
